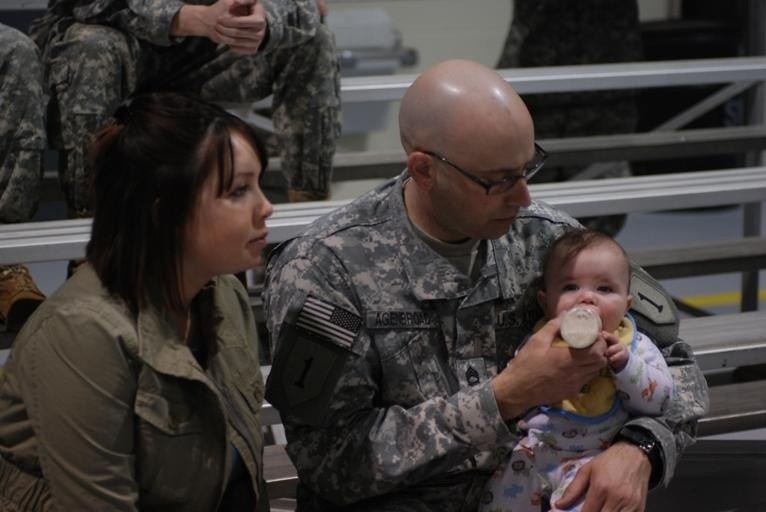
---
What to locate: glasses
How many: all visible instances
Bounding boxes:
[411,142,548,196]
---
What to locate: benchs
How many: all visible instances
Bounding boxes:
[0,0,766,511]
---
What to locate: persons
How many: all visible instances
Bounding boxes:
[260,52,713,510]
[0,2,143,226]
[0,91,277,509]
[61,1,346,219]
[481,226,679,512]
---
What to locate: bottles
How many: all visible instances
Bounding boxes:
[560,306,603,350]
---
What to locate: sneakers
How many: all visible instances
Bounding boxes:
[286,187,332,204]
[0,265,47,330]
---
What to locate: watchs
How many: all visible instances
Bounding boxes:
[611,422,668,490]
